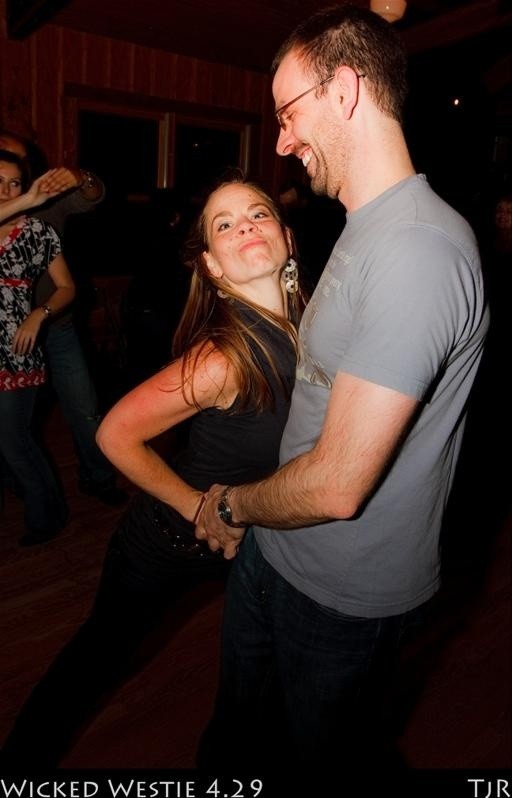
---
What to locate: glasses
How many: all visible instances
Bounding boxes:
[272,74,366,132]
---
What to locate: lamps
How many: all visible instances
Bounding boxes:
[369,0,407,23]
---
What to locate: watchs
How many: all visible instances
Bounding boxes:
[40,303,51,319]
[218,486,254,528]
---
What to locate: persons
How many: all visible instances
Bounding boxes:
[494,196,512,245]
[0,149,77,546]
[130,168,192,259]
[0,134,130,507]
[0,177,298,797]
[196,3,492,798]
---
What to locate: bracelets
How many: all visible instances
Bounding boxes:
[79,169,96,189]
[192,496,205,524]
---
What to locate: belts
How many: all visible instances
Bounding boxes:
[132,497,220,561]
